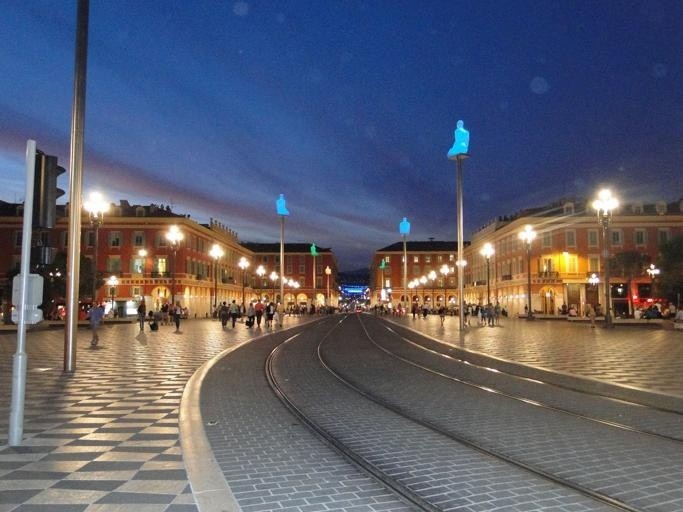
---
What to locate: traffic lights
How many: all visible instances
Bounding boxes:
[30,245,59,266]
[32,153,66,230]
[39,228,67,254]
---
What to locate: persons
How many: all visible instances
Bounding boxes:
[211,298,340,330]
[147,299,190,334]
[85,300,104,347]
[136,299,145,331]
[43,294,106,330]
[338,295,683,328]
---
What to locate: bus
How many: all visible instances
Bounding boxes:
[354,303,361,313]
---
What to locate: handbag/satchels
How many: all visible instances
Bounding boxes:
[150,324,157,330]
[246,321,253,326]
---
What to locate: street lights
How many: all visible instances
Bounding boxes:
[375,215,496,324]
[588,188,617,329]
[646,262,658,308]
[81,190,109,306]
[276,192,290,317]
[518,224,537,323]
[162,224,185,306]
[138,249,147,302]
[587,272,602,312]
[107,275,118,310]
[445,119,471,332]
[204,241,331,315]
